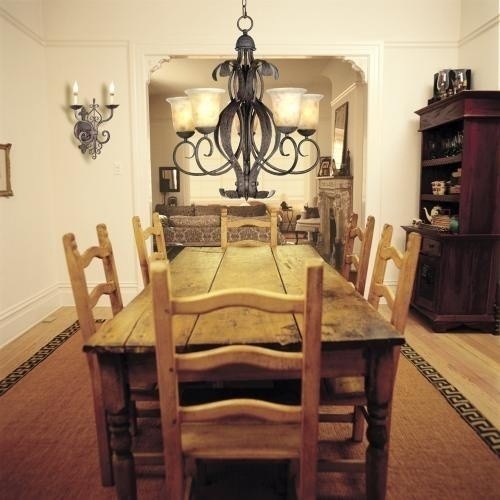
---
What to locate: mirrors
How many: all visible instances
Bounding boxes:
[333,102,348,170]
[159,166,180,191]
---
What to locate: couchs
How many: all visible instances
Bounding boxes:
[154,205,285,246]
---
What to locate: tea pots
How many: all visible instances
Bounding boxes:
[422,204,443,222]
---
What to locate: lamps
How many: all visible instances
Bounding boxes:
[165,1,324,200]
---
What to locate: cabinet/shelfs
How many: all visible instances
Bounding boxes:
[399,90,499,334]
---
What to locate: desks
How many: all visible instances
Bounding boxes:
[82,246,406,498]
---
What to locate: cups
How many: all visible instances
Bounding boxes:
[432,181,446,195]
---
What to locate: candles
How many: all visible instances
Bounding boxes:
[71,81,114,106]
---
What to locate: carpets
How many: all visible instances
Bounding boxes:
[1,319,500,498]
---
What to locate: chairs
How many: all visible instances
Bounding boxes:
[320,214,421,473]
[133,214,168,286]
[150,260,324,498]
[295,206,321,245]
[220,209,278,246]
[64,223,166,487]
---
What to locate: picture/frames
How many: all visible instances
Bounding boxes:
[0,144,14,197]
